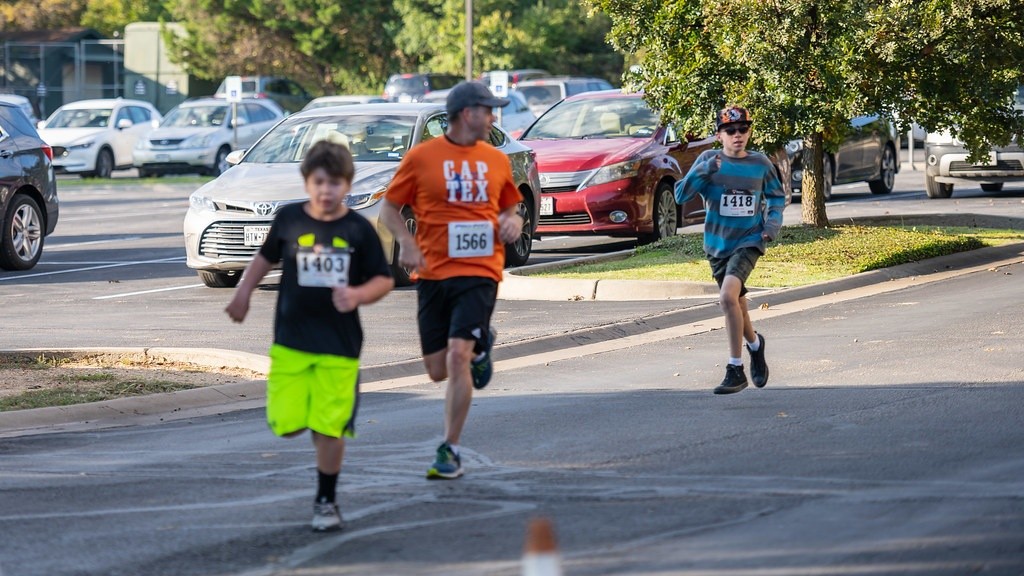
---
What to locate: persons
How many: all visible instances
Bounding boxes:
[221,141,393,523]
[677,107,786,393]
[382,82,523,479]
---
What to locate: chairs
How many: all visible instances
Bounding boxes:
[367,136,394,153]
[600,112,623,137]
[394,135,410,157]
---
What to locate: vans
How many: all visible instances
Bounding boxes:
[923,82,1024,198]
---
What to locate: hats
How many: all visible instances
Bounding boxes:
[446,81,510,114]
[718,105,753,132]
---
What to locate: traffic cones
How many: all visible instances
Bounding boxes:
[521,517,562,576]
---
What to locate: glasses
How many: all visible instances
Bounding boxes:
[721,125,750,136]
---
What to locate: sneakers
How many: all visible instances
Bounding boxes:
[471,326,497,390]
[310,502,344,530]
[427,441,464,479]
[714,363,748,394]
[746,330,769,387]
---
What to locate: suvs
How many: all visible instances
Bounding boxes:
[35,99,163,178]
[784,114,902,201]
[0,93,59,272]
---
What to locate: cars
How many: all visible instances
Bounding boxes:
[131,93,288,178]
[183,69,612,287]
[515,89,791,246]
